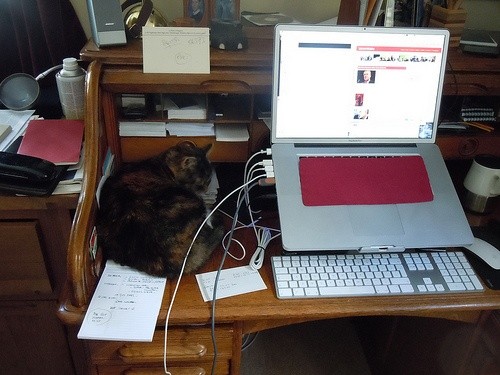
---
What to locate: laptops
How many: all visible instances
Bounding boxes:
[269,24,476,250]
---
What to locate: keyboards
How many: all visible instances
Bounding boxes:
[271,251,485,300]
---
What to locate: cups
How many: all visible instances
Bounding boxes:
[462,153,500,215]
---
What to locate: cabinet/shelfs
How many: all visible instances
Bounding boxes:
[0,194,87,375]
[58,25,500,375]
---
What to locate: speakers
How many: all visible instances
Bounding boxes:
[87,0,127,47]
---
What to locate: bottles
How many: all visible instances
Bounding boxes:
[56,58,86,120]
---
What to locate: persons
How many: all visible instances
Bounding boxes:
[355,109,368,120]
[191,0,202,20]
[361,71,374,83]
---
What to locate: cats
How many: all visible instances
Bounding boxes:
[94,140,226,279]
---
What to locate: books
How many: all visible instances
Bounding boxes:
[0,120,85,165]
